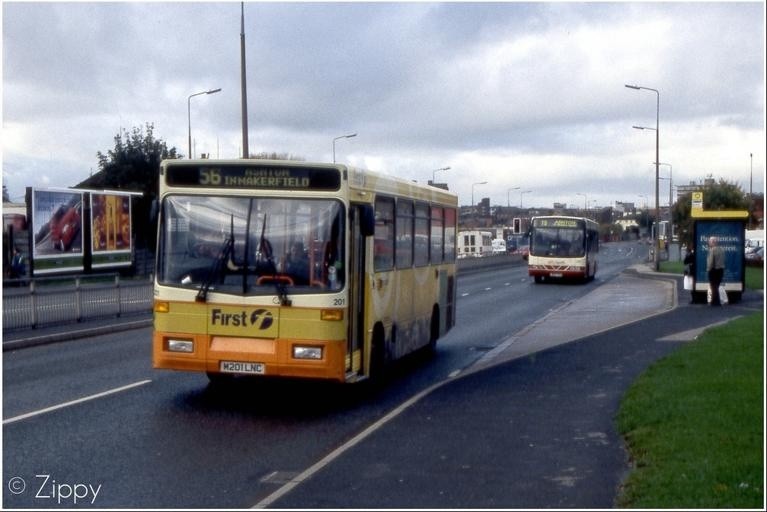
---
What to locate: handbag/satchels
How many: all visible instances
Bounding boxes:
[707,285,728,304]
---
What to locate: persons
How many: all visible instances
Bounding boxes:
[707,236,728,308]
[684,244,696,304]
[285,242,309,288]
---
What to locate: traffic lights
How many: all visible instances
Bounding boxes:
[512,217,521,235]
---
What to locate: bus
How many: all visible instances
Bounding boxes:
[520,215,600,285]
[148,152,466,390]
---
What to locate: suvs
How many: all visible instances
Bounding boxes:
[47,203,80,250]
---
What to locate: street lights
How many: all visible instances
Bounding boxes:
[187,87,222,160]
[626,82,675,270]
[507,186,521,233]
[332,132,358,163]
[471,179,488,229]
[520,190,534,219]
[432,164,451,185]
[748,151,754,230]
[575,192,599,214]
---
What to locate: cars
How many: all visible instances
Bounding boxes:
[521,248,529,260]
[397,233,451,253]
[745,238,765,261]
